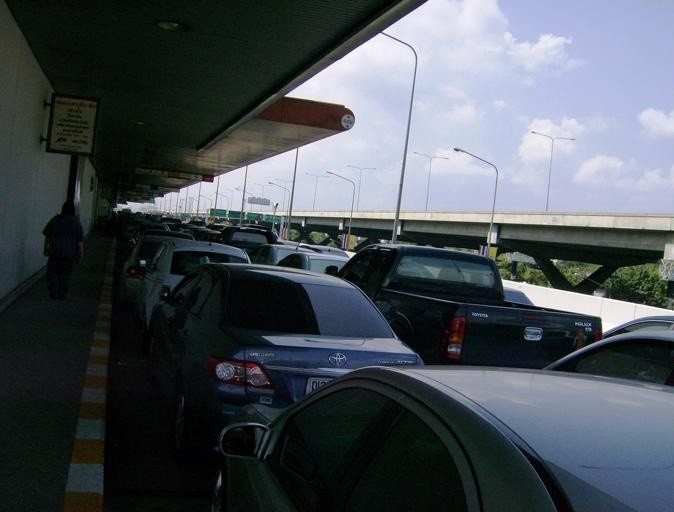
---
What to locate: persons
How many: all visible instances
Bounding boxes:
[41,202,83,299]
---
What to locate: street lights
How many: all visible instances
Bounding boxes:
[348,165,376,212]
[454,146,498,258]
[531,131,576,213]
[326,171,355,251]
[182,188,261,224]
[414,151,449,213]
[269,181,290,240]
[306,172,329,212]
